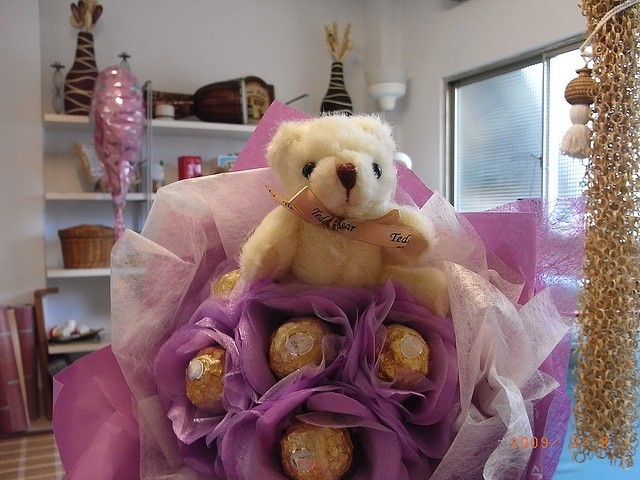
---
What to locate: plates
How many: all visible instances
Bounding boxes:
[48,327,104,344]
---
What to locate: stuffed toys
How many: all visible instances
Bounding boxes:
[237,111,452,319]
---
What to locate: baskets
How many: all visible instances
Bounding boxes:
[57,224,114,269]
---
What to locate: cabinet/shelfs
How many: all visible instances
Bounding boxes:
[43,79,258,433]
